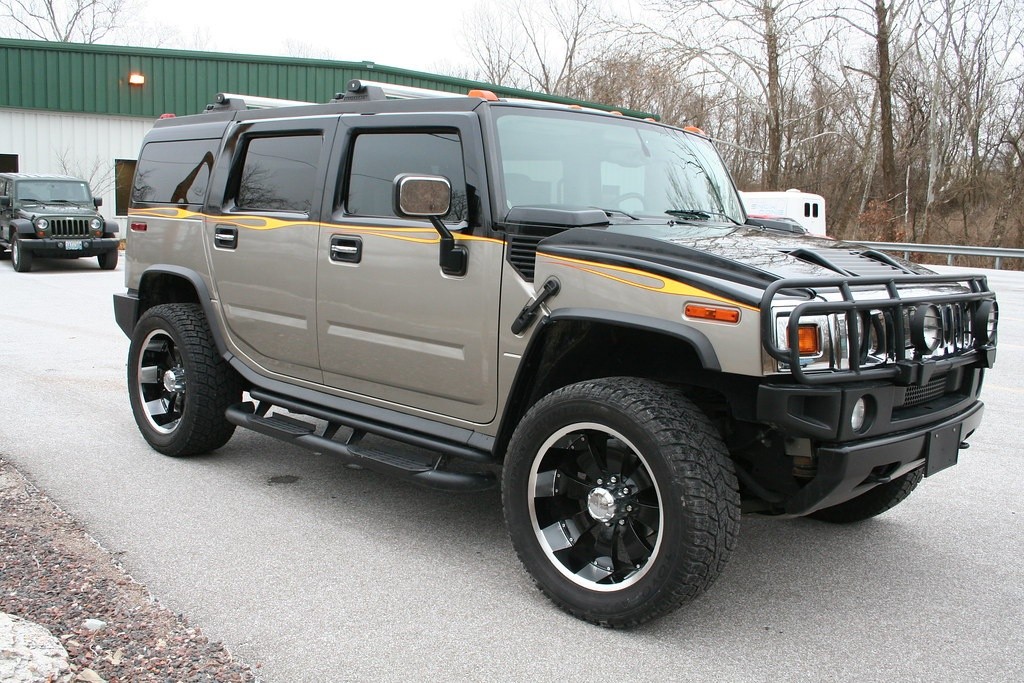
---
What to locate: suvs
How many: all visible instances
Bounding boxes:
[1,173,121,273]
[114,77,1003,630]
